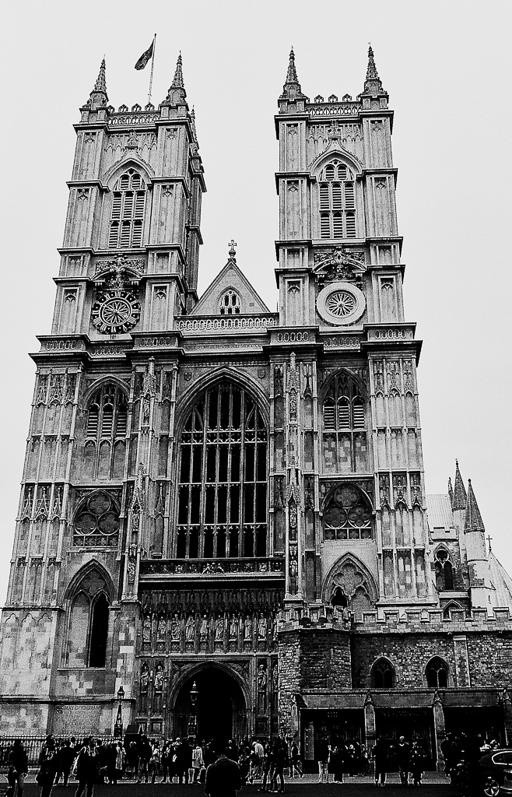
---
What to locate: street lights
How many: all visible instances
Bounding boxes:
[187,681,199,746]
[114,686,125,736]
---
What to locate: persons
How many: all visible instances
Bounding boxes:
[7,731,511,797]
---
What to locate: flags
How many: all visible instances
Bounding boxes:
[134,37,154,70]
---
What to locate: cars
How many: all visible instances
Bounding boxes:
[451,749,512,797]
